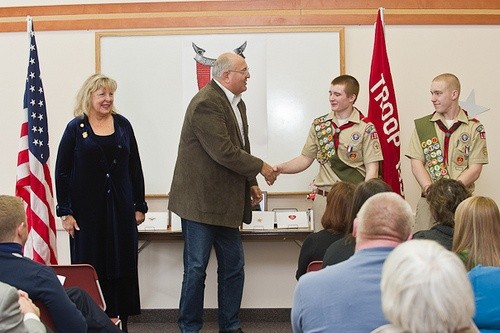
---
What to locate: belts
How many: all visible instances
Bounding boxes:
[316,186,329,196]
[420,190,426,197]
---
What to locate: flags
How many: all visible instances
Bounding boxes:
[368,8,406,198]
[14,16,60,266]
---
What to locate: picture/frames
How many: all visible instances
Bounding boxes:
[95,26,346,200]
[251,191,267,211]
[272,208,298,224]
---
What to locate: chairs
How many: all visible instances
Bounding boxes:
[46,264,123,331]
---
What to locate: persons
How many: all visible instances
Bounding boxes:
[451,195,500,333]
[290,192,416,333]
[295,181,360,281]
[370,237,481,333]
[0,282,48,333]
[410,177,473,253]
[55,73,149,333]
[0,194,125,332]
[318,178,392,269]
[265,74,384,235]
[403,72,489,235]
[168,52,277,333]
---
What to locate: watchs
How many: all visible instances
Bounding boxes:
[61,214,71,221]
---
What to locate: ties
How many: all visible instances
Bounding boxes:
[432,109,468,166]
[328,117,356,156]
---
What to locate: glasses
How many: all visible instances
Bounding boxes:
[228,67,250,74]
